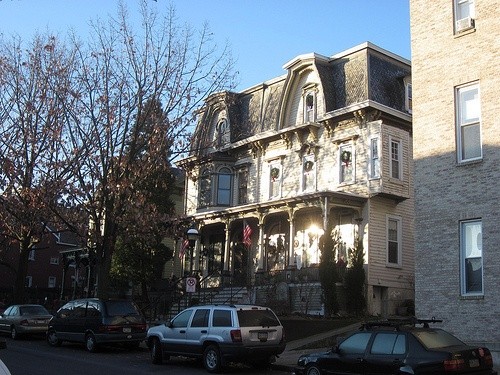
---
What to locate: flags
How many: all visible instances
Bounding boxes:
[243,219,253,247]
[179,234,189,260]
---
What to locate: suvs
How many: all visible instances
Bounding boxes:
[146,302,286,372]
[46,296,146,352]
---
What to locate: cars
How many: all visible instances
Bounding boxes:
[0,304,54,341]
[297,315,498,375]
